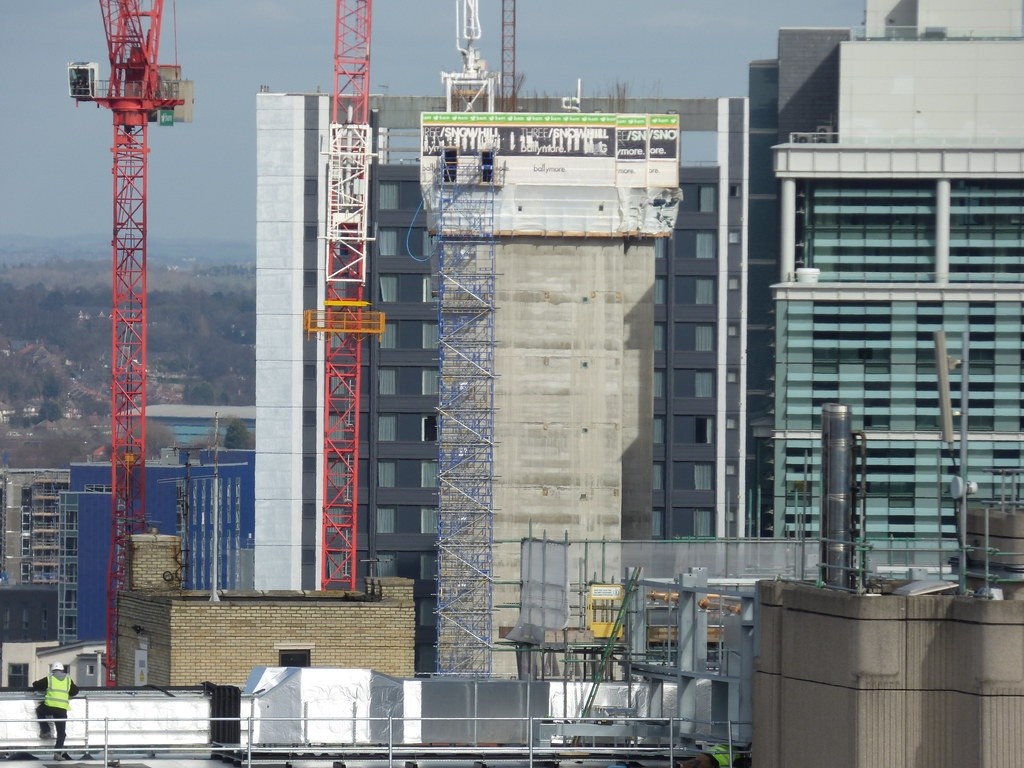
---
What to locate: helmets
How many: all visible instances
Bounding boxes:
[51,662,65,672]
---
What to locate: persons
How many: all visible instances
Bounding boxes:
[31,662,81,761]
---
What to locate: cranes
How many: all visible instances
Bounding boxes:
[422,1,502,681]
[303,2,389,592]
[68,2,192,690]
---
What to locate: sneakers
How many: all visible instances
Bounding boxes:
[53,753,66,761]
[39,732,51,739]
